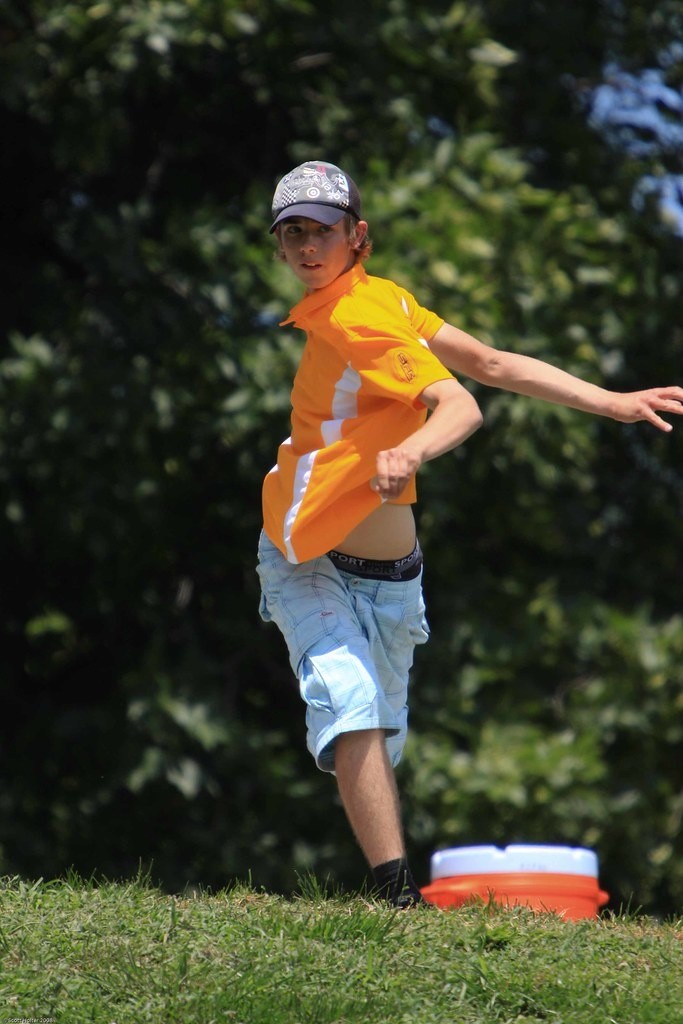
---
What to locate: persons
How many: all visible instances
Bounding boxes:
[255,161,683,907]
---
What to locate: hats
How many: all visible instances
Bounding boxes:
[268,160,361,234]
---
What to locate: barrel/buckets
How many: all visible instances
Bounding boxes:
[420,843,611,922]
